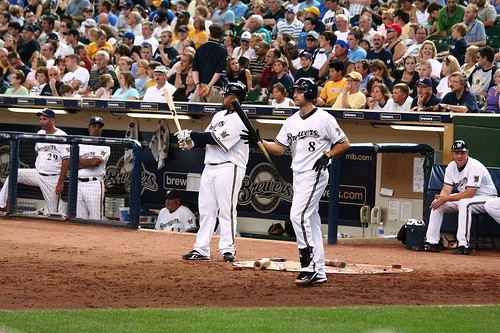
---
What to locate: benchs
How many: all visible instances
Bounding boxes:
[424,164,500,250]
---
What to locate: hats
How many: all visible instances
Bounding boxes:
[386,23,402,36]
[335,39,349,54]
[284,5,294,14]
[119,33,135,40]
[299,51,312,61]
[417,76,433,87]
[164,189,182,199]
[36,108,56,118]
[306,30,319,41]
[154,65,167,73]
[177,24,189,32]
[175,10,190,19]
[83,5,93,12]
[83,18,96,28]
[89,116,105,127]
[345,71,362,81]
[152,0,169,10]
[240,30,252,42]
[21,24,35,32]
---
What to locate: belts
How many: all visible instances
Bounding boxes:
[78,176,98,182]
[38,172,59,176]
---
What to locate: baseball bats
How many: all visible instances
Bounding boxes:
[231,99,273,165]
[163,89,189,151]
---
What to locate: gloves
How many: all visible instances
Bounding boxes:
[239,127,263,145]
[174,128,193,140]
[178,138,195,151]
[312,152,330,171]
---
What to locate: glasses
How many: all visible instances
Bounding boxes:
[345,77,356,83]
[387,29,394,33]
[255,44,263,48]
[306,36,316,41]
[25,14,34,18]
[415,32,426,35]
[381,16,389,20]
[221,93,228,98]
[60,24,66,28]
[47,73,59,76]
[406,62,414,65]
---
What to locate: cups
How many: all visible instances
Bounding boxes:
[118,206,130,222]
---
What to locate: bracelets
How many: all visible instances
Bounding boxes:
[447,104,448,109]
[196,82,200,85]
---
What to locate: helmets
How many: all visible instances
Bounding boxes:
[292,76,318,99]
[219,82,247,102]
[451,139,468,152]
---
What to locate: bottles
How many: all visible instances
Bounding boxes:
[378,221,385,238]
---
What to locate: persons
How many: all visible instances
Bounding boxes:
[114,2,135,29]
[419,140,498,255]
[192,6,213,35]
[391,83,414,111]
[436,0,481,39]
[210,0,235,31]
[48,66,63,96]
[366,33,393,74]
[484,198,500,225]
[192,23,228,103]
[131,44,152,77]
[263,0,285,33]
[53,58,68,80]
[86,29,113,65]
[281,0,301,14]
[37,16,66,46]
[134,59,151,100]
[249,32,266,60]
[67,116,112,220]
[7,51,32,82]
[427,1,444,35]
[111,73,139,100]
[297,17,320,57]
[239,77,351,286]
[367,83,393,110]
[370,0,382,31]
[42,42,55,68]
[467,46,499,99]
[152,30,181,73]
[143,65,177,102]
[16,25,40,68]
[172,46,196,69]
[37,0,68,29]
[133,4,147,19]
[365,76,384,109]
[389,1,397,11]
[358,16,377,47]
[397,0,416,23]
[80,8,94,27]
[4,35,18,52]
[0,108,71,216]
[0,48,12,86]
[392,9,409,41]
[87,28,98,45]
[29,66,52,96]
[0,0,9,11]
[61,29,86,57]
[96,12,118,36]
[332,71,367,109]
[93,0,119,26]
[170,27,196,55]
[155,190,198,232]
[228,0,248,18]
[22,0,42,25]
[172,72,196,102]
[195,0,212,20]
[298,0,321,11]
[299,30,320,65]
[110,2,117,14]
[59,84,74,97]
[382,24,407,61]
[74,45,93,73]
[22,51,47,91]
[74,50,120,98]
[63,0,92,30]
[57,19,72,43]
[276,6,305,43]
[173,83,249,262]
[377,9,393,44]
[480,69,500,114]
[316,61,348,108]
[321,0,350,31]
[61,54,90,97]
[100,24,117,46]
[389,56,420,96]
[87,74,115,99]
[224,34,236,60]
[187,16,209,50]
[436,23,467,67]
[361,60,394,98]
[0,66,9,94]
[293,51,326,85]
[149,0,176,26]
[4,70,29,96]
[268,58,293,105]
[134,22,160,55]
[246,15,272,44]
[124,11,143,36]
[394,28,428,71]
[167,52,194,89]
[347,30,367,63]
[114,44,130,72]
[22,10,43,40]
[311,32,337,70]
[469,0,497,27]
[272,82,296,106]
[0,11,10,41]
[232,31,255,68]
[116,56,132,82]
[0,39,4,48]
[460,45,479,78]
[249,48,289,91]
[169,10,194,43]
[414,0,435,30]
[354,60,372,90]
[334,14,350,43]
[145,63,160,90]
[122,32,141,70]
[249,41,271,88]
[329,7,345,33]
[269,33,294,60]
[462,4,487,46]
[416,40,442,78]
[358,40,370,52]
[296,11,304,23]
[318,40,355,85]
[433,71,478,113]
[409,76,441,112]
[8,22,23,41]
[152,12,171,44]
[411,61,439,98]
[45,33,74,58]
[79,18,96,45]
[490,0,500,14]
[241,0,268,31]
[9,6,25,27]
[170,0,194,28]
[436,55,464,100]
[401,25,419,49]
[226,58,254,97]
[365,3,389,21]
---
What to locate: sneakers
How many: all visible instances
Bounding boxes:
[309,273,328,283]
[182,249,210,261]
[420,242,440,252]
[454,245,472,254]
[223,252,234,262]
[294,270,318,285]
[46,32,58,40]
